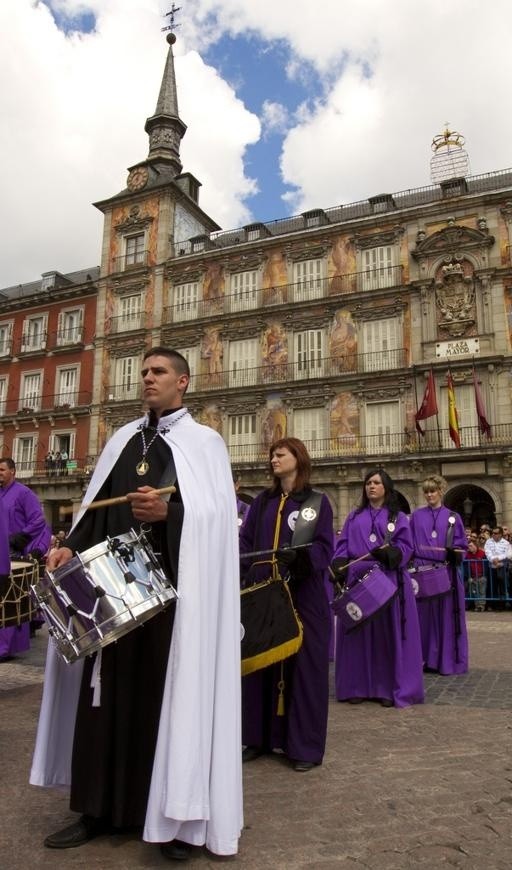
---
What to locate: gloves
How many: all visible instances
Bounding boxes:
[369,548,388,563]
[9,532,42,559]
[275,543,298,567]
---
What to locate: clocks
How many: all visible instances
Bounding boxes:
[126,166,148,193]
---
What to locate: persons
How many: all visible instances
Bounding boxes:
[28,346,245,860]
[0,457,51,663]
[239,438,333,773]
[231,473,251,538]
[50,530,71,549]
[462,522,512,611]
[328,469,425,708]
[408,475,469,676]
[40,447,69,478]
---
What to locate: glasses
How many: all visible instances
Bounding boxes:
[491,532,499,535]
[479,537,485,540]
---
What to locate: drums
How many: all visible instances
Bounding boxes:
[408,563,455,602]
[27,528,179,666]
[331,564,400,635]
[241,579,303,676]
[0,555,39,629]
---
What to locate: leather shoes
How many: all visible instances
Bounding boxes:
[243,746,272,762]
[44,819,109,848]
[160,840,193,860]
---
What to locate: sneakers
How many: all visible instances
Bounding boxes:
[382,698,393,707]
[293,760,312,771]
[350,697,363,704]
[474,607,500,612]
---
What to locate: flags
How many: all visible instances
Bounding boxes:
[445,373,463,451]
[410,368,438,437]
[469,367,491,438]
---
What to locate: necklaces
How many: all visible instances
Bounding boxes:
[430,506,442,539]
[136,410,188,476]
[369,504,383,543]
